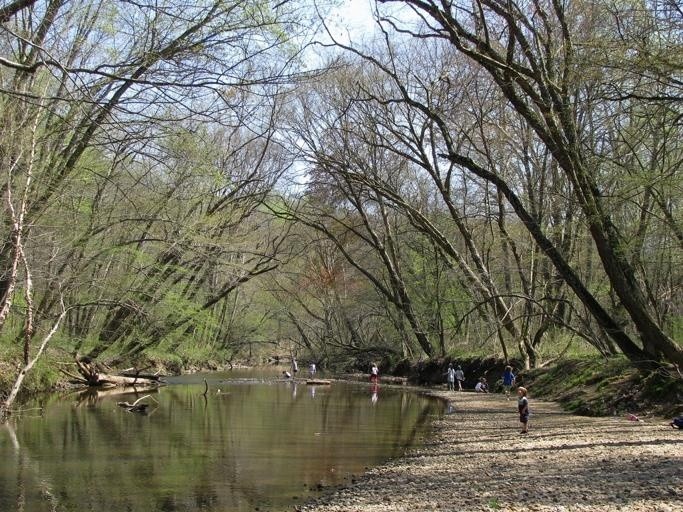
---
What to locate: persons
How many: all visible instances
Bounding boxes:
[441,362,490,395]
[291,358,298,378]
[308,361,316,378]
[517,386,529,435]
[369,363,379,385]
[500,365,517,401]
[282,371,292,379]
